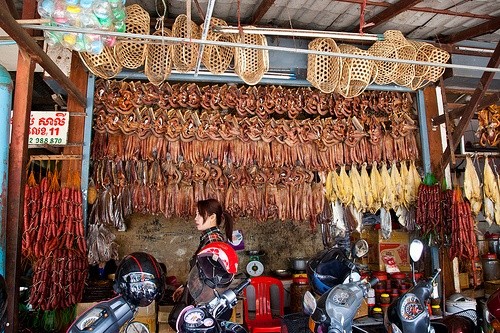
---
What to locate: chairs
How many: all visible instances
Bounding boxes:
[244,276,287,333]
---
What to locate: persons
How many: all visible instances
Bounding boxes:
[172,199,233,306]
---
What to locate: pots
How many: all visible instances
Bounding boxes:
[286,257,311,270]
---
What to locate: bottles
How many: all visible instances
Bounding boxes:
[470,256,484,288]
[361,271,441,317]
[290,276,315,312]
[488,233,500,253]
[459,258,470,291]
[483,253,500,280]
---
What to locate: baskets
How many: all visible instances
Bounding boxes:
[144,27,173,86]
[235,24,269,86]
[339,29,452,99]
[113,6,151,69]
[175,14,199,72]
[200,17,236,74]
[78,42,122,80]
[306,37,343,95]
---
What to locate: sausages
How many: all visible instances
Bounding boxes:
[22,178,88,310]
[415,185,478,288]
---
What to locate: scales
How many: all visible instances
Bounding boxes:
[242,249,265,279]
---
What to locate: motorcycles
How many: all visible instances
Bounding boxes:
[65,251,167,333]
[280,236,500,333]
[167,242,253,333]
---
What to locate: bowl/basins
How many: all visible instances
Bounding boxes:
[272,269,289,276]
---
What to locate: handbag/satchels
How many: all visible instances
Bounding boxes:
[168,286,197,331]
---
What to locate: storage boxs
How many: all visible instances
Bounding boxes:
[230,299,243,325]
[76,300,176,333]
[349,229,411,271]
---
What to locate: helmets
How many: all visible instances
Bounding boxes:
[307,249,352,295]
[116,252,164,306]
[196,240,239,287]
